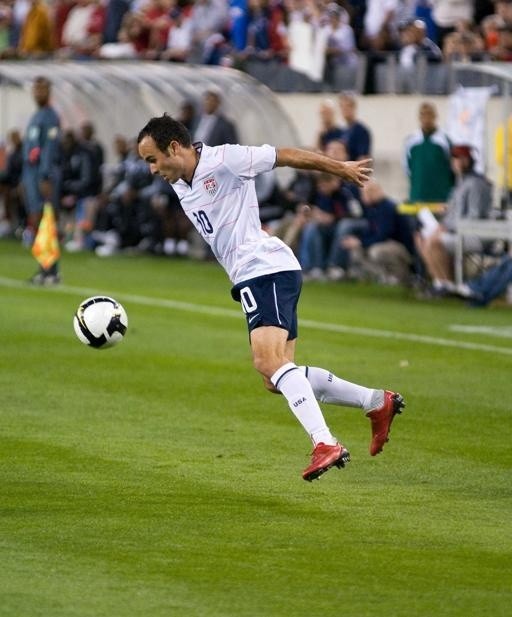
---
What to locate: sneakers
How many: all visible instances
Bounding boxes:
[303,442,349,481]
[366,390,405,454]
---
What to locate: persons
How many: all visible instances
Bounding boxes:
[255,96,512,307]
[0,76,239,287]
[137,111,405,482]
[0,0,512,94]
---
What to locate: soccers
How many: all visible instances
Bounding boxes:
[73,295,127,349]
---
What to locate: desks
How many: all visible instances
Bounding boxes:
[455,218,512,284]
